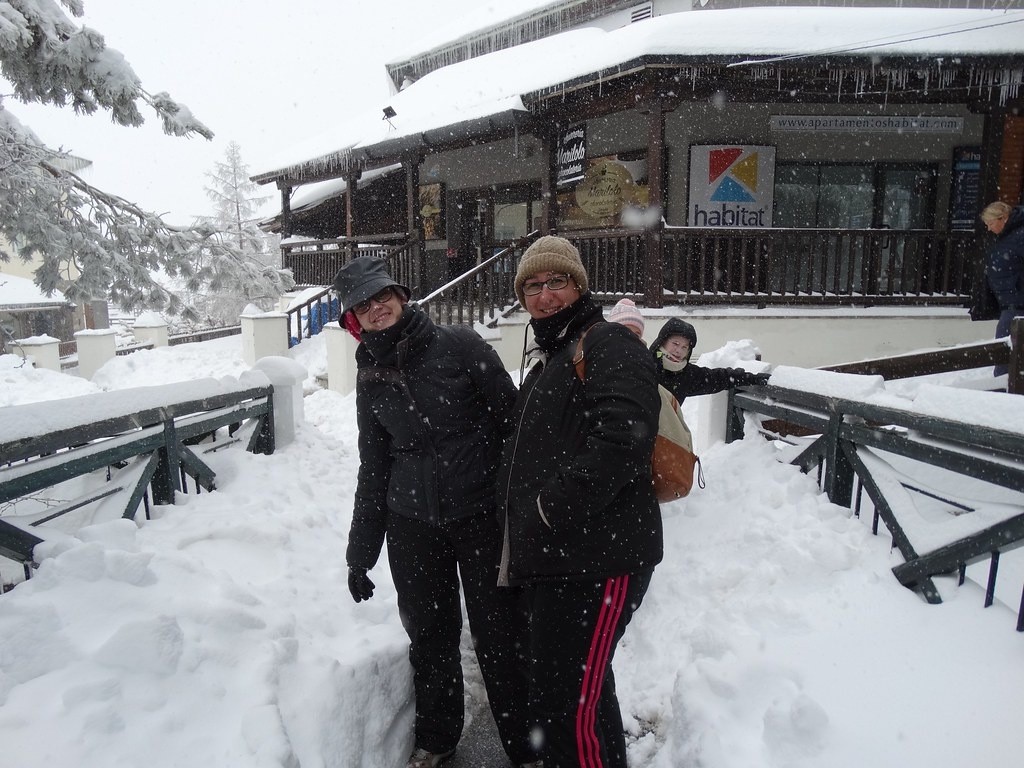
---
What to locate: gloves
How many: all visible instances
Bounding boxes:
[347,564,376,603]
[745,372,771,386]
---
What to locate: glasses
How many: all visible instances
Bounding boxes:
[352,287,392,314]
[521,273,575,296]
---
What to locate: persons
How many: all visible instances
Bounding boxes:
[981,201,1024,393]
[333,258,519,768]
[494,234,662,768]
[605,298,771,407]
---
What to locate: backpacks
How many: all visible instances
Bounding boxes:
[572,320,706,503]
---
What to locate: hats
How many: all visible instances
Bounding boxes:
[514,234,588,314]
[333,256,412,328]
[607,298,645,338]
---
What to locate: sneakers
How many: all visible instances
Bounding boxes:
[521,759,562,768]
[400,747,457,768]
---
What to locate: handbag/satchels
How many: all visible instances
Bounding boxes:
[968,291,1001,321]
